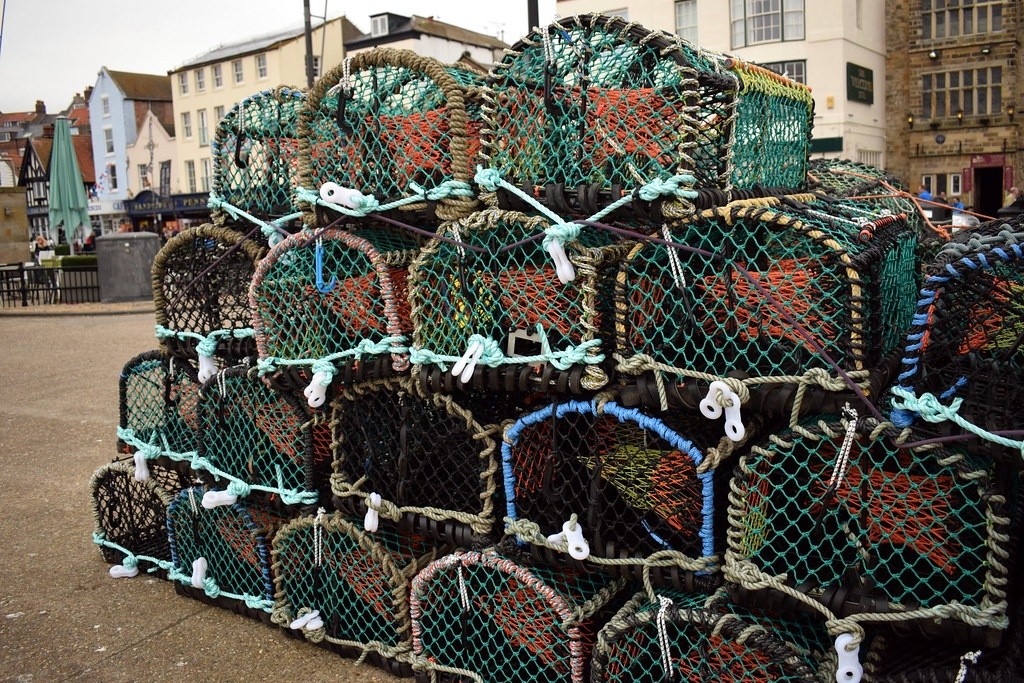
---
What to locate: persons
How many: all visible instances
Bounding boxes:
[938,191,949,204]
[951,197,964,211]
[916,185,934,202]
[912,192,918,197]
[1004,186,1019,208]
[34,236,49,262]
[966,206,973,211]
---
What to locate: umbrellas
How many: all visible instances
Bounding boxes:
[50,115,93,255]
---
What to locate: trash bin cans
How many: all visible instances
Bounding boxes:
[920,202,954,237]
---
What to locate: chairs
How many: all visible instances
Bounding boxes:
[0,263,57,301]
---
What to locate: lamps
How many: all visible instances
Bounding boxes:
[981,47,991,54]
[955,108,964,123]
[1007,104,1016,121]
[928,51,937,60]
[905,111,913,130]
